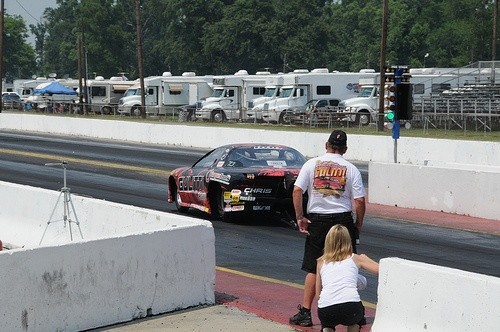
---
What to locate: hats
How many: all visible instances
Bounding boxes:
[328,130,347,145]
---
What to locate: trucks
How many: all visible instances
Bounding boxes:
[18,68,500,127]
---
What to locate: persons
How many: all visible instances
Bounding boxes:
[57,101,65,112]
[308,104,318,127]
[305,101,311,114]
[316,224,378,332]
[289,130,367,326]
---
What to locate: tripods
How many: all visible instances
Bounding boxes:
[37,161,84,247]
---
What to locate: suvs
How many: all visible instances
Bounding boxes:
[2,92,23,109]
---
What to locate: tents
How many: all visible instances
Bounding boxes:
[33,81,78,114]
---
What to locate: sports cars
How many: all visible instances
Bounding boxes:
[167,143,308,223]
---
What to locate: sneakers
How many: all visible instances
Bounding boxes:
[289,304,312,327]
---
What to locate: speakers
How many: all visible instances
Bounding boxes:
[395,82,413,120]
